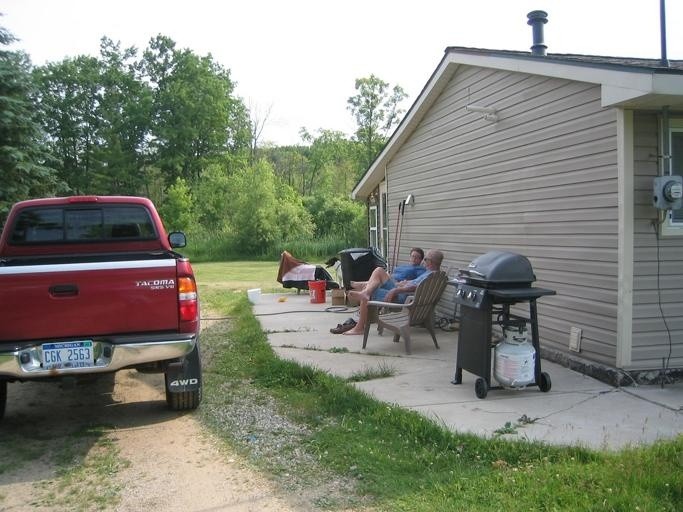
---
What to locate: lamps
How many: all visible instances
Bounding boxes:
[404,193,414,205]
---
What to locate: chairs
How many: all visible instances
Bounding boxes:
[361,269,449,357]
[280,250,332,294]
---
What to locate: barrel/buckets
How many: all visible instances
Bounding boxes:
[308,280,327,304]
[247,288,262,304]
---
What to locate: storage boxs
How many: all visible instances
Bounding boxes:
[330,287,346,306]
[344,289,359,308]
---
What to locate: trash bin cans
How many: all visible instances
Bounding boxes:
[337,247,376,301]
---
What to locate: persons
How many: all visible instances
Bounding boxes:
[342,248,444,335]
[350,248,427,289]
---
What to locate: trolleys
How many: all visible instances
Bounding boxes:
[444,246,555,399]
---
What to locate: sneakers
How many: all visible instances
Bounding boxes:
[330,318,357,334]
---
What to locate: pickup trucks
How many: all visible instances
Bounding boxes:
[0,190,207,416]
